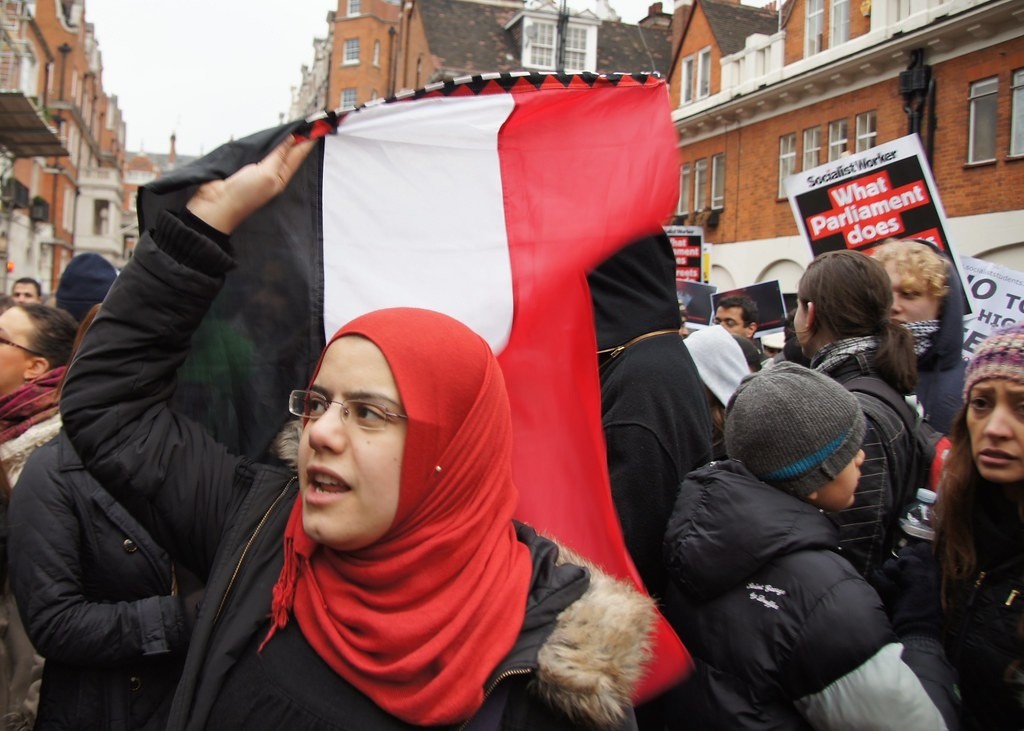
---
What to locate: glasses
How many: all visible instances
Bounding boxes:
[714,320,748,329]
[289,389,407,430]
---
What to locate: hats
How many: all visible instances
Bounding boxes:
[56,253,117,321]
[963,321,1024,403]
[724,361,867,498]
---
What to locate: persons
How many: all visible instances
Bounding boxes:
[678,239,968,581]
[586,226,713,731]
[60,134,653,731]
[661,362,962,731]
[0,252,207,731]
[937,325,1024,731]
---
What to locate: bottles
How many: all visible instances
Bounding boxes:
[890,487,938,559]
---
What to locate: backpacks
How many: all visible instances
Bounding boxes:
[843,376,954,563]
